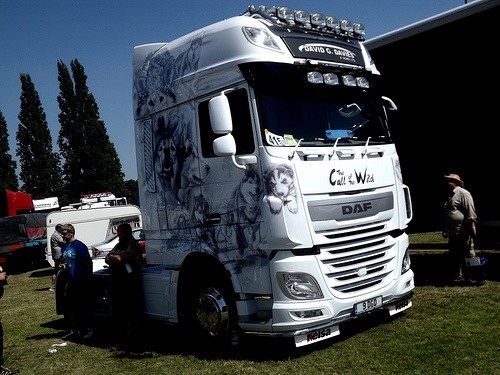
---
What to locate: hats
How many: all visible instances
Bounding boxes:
[444,173,464,187]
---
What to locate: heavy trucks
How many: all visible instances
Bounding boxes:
[1,3,416,362]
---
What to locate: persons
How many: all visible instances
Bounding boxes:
[105,224,143,344]
[60,224,94,338]
[51,224,64,288]
[442,173,484,285]
[0,267,12,375]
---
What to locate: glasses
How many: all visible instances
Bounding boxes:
[61,232,68,235]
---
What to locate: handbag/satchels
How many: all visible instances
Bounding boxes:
[467,234,488,280]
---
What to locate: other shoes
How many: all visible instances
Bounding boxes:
[476,279,485,286]
[84,327,96,339]
[110,342,122,352]
[52,285,55,289]
[452,277,466,283]
[61,329,80,339]
[0,366,13,375]
